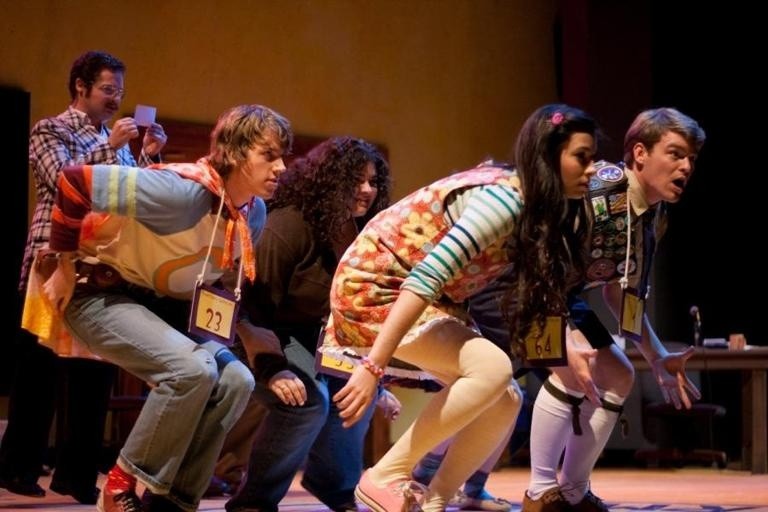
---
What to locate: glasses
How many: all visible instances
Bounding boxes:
[88,80,128,98]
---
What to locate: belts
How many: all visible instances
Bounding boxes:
[76,262,154,304]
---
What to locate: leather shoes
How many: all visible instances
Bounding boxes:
[0,473,45,497]
[204,475,231,495]
[49,472,99,505]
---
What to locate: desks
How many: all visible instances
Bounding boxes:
[607,343,768,475]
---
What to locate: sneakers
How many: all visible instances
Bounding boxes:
[457,491,511,512]
[571,490,608,512]
[301,479,359,512]
[96,482,144,512]
[225,497,278,512]
[521,486,569,512]
[358,469,426,512]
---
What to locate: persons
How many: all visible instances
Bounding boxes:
[223,137,402,512]
[3,54,169,506]
[331,107,595,512]
[522,109,700,512]
[45,106,288,511]
